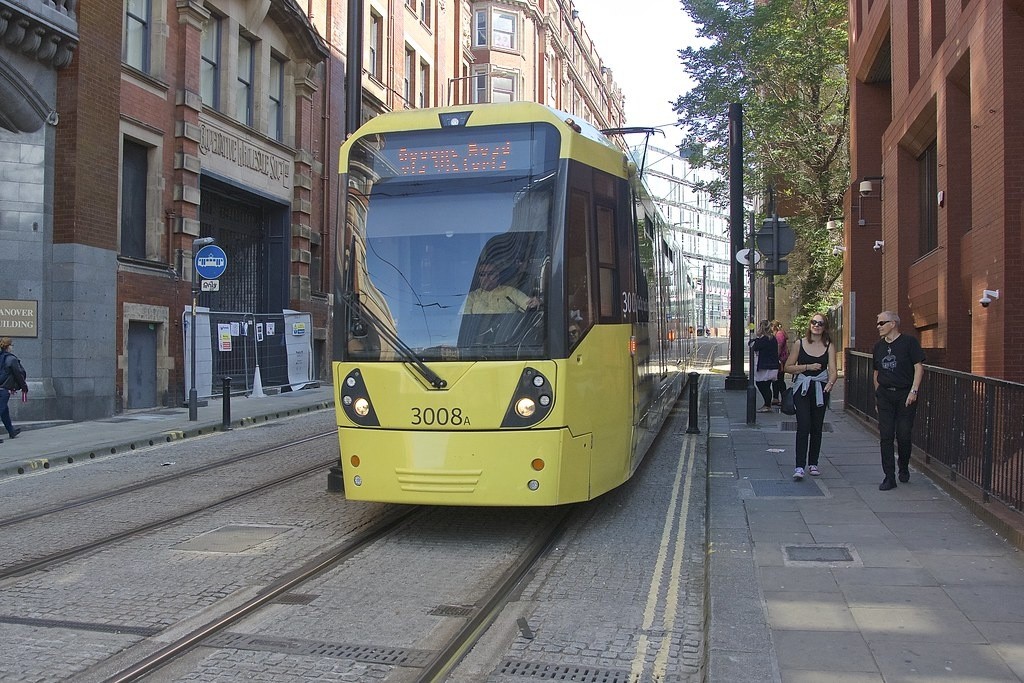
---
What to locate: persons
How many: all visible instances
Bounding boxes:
[456,260,544,315]
[747,319,790,412]
[872,311,926,490]
[784,313,837,478]
[0,336,28,444]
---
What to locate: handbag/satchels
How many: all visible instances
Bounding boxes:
[781,388,800,416]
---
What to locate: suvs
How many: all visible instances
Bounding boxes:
[697,325,712,336]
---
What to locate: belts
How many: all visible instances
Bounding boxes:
[885,387,903,391]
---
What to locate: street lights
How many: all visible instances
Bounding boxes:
[673,220,692,242]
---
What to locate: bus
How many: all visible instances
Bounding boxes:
[326,99,697,515]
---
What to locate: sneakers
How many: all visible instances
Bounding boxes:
[898,462,910,483]
[879,477,897,490]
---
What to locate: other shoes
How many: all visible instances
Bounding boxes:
[793,467,805,478]
[809,465,820,475]
[0,439,3,443]
[771,399,780,406]
[756,406,771,413]
[10,428,21,439]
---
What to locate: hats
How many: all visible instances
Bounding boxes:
[0,338,13,349]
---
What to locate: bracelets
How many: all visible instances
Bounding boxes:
[910,390,919,395]
[805,364,808,370]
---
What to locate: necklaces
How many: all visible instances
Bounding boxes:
[884,333,901,343]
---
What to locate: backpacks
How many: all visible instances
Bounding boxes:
[5,353,26,390]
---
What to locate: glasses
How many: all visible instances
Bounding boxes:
[810,320,825,327]
[876,321,892,328]
[479,271,497,277]
